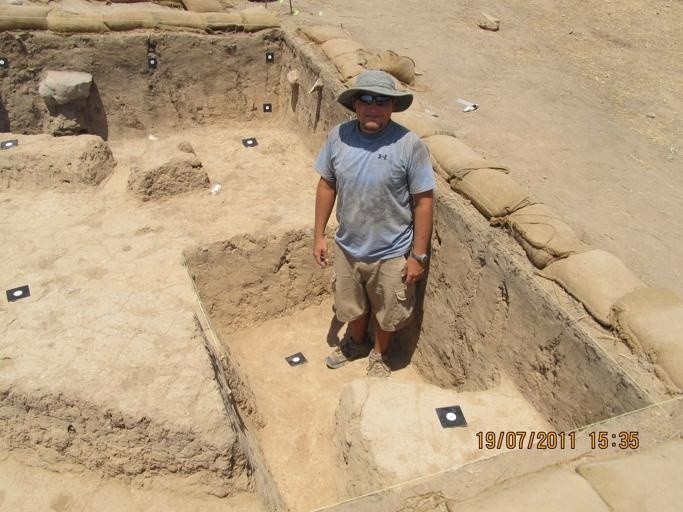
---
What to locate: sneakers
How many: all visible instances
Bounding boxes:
[367,350,391,377]
[326,336,372,369]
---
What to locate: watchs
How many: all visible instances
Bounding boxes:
[411,251,428,264]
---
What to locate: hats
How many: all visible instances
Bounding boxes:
[338,70,413,111]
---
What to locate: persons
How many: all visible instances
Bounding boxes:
[311,68,437,378]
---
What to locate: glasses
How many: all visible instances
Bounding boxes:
[359,94,391,104]
